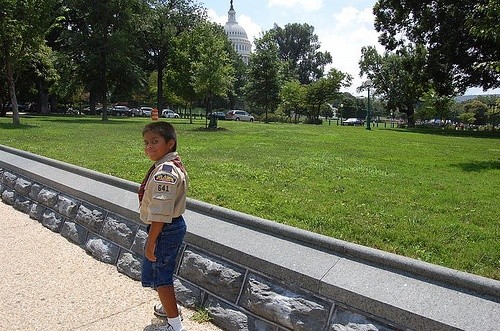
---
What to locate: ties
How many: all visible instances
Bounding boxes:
[138,165,156,202]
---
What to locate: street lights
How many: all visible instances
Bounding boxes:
[365,78,372,131]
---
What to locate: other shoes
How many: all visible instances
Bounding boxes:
[159,323,187,331]
[154,304,182,320]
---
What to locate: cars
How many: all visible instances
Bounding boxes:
[208,112,225,120]
[84,105,180,118]
[225,110,254,122]
[342,118,364,126]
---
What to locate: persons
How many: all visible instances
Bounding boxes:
[136,120,191,330]
